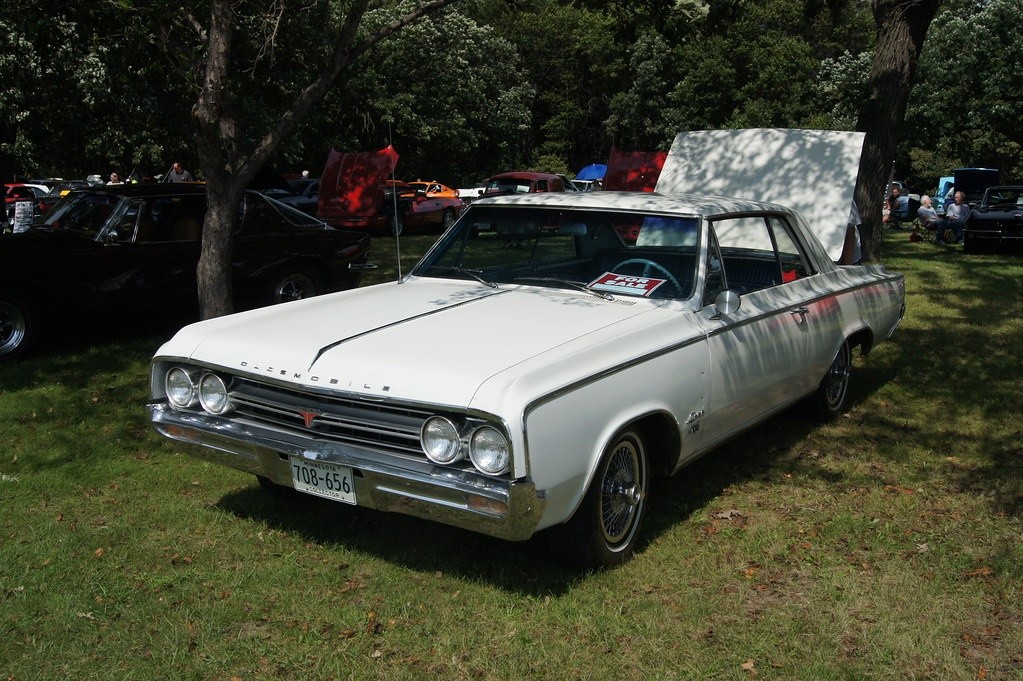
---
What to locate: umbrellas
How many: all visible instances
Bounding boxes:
[574,163,608,189]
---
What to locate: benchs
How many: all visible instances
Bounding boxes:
[589,249,778,307]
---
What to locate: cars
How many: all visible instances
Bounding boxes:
[147,124,906,570]
[30,177,68,190]
[1,179,374,360]
[3,184,63,214]
[883,168,1023,251]
[386,180,460,201]
[263,176,322,210]
[477,173,566,200]
[555,173,604,193]
[318,178,465,237]
[46,180,93,201]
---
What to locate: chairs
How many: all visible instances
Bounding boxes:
[917,212,952,242]
[892,198,921,228]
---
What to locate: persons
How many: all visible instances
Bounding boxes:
[166,163,194,183]
[105,172,124,186]
[835,198,864,266]
[945,190,970,223]
[917,195,965,246]
[888,187,910,230]
